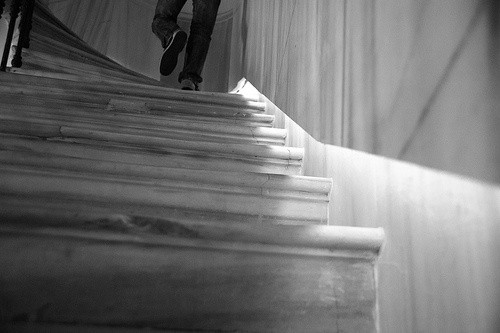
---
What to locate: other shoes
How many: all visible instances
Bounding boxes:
[179,77,200,92]
[159,28,187,76]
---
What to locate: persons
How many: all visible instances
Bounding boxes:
[150,0,223,93]
